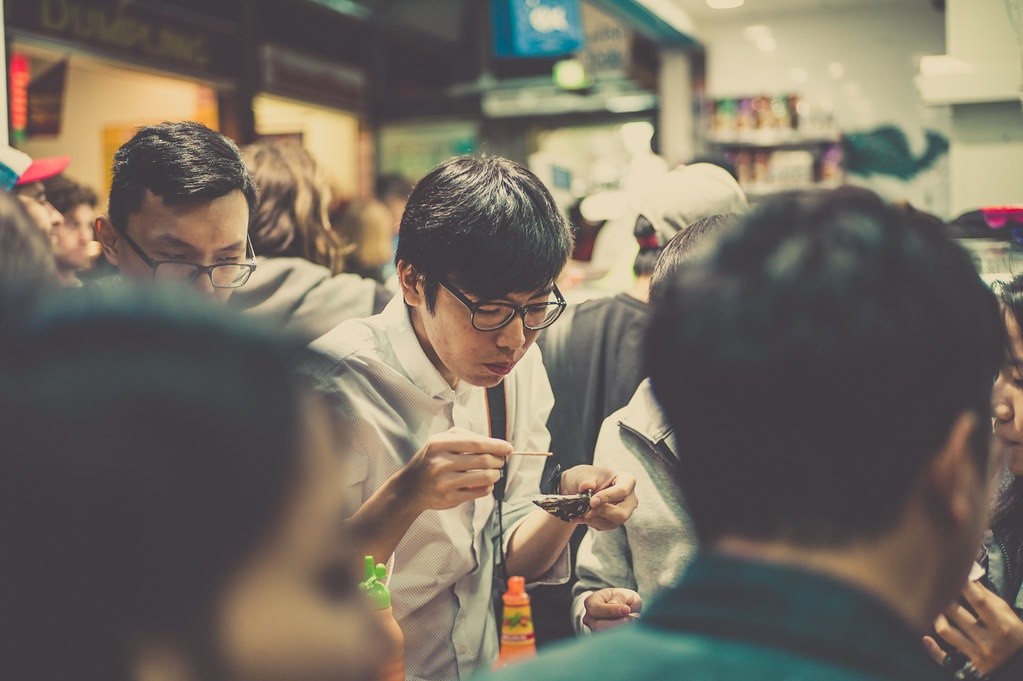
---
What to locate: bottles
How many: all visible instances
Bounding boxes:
[716,97,792,130]
[722,144,843,184]
[498,578,536,668]
[356,556,404,681]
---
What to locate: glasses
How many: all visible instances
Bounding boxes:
[111,219,257,288]
[434,273,567,332]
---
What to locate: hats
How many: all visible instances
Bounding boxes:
[0,144,70,195]
[631,162,750,248]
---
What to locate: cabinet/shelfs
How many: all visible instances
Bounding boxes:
[706,132,856,200]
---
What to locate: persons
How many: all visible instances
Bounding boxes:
[530,162,747,496]
[920,272,1023,681]
[0,132,419,346]
[0,284,403,681]
[92,121,259,308]
[455,186,1011,681]
[567,210,747,639]
[302,155,640,681]
